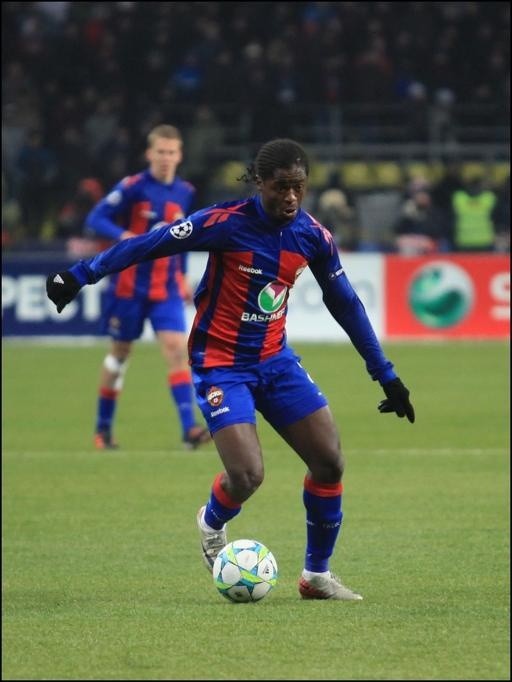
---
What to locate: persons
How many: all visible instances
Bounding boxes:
[1,2,511,255]
[45,137,415,602]
[81,122,213,452]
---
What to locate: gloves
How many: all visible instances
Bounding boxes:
[378,377,416,424]
[46,269,82,313]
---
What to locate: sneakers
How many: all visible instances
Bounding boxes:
[95,430,117,449]
[186,426,211,446]
[298,570,363,602]
[196,505,228,573]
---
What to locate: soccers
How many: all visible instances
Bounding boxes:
[213,539,278,602]
